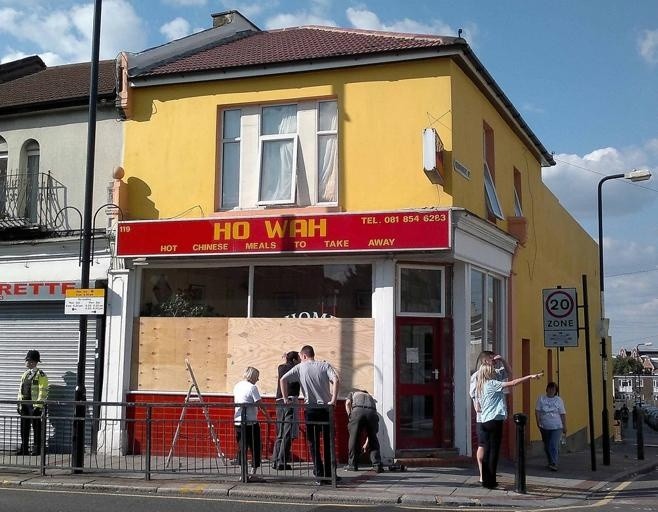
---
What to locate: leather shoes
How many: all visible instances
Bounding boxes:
[344,465,358,471]
[272,464,290,469]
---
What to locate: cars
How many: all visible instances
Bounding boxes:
[634,397,658,430]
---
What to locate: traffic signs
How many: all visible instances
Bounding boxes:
[541,287,579,349]
[63,288,105,316]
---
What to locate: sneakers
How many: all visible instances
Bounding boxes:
[238,475,266,482]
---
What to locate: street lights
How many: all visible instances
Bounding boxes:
[628,371,642,408]
[636,342,653,407]
[593,168,652,467]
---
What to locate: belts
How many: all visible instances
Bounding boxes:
[353,406,375,409]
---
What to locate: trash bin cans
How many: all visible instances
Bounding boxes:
[614,421,622,442]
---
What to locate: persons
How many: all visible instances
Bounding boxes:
[232,365,270,483]
[468,349,513,484]
[534,382,568,473]
[14,349,51,456]
[343,388,385,473]
[475,363,545,488]
[271,350,302,470]
[279,344,343,486]
